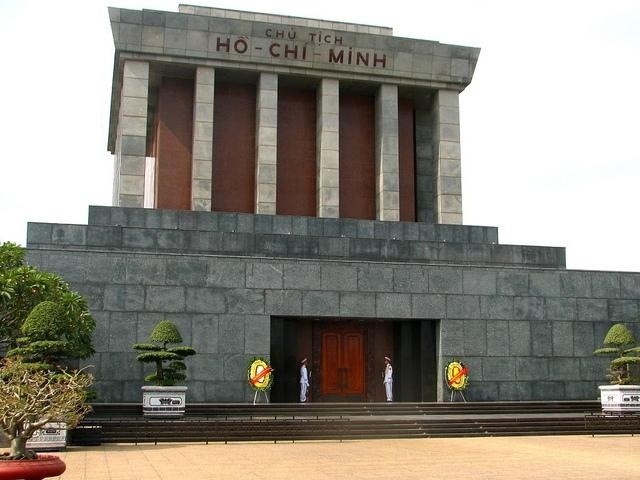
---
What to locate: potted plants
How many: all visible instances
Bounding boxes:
[132,320,198,418]
[7,300,72,451]
[590,322,640,412]
[0,357,96,479]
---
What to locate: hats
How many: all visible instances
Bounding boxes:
[385,356,391,362]
[301,358,308,363]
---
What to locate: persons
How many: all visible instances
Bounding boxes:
[299,358,310,403]
[383,356,393,402]
